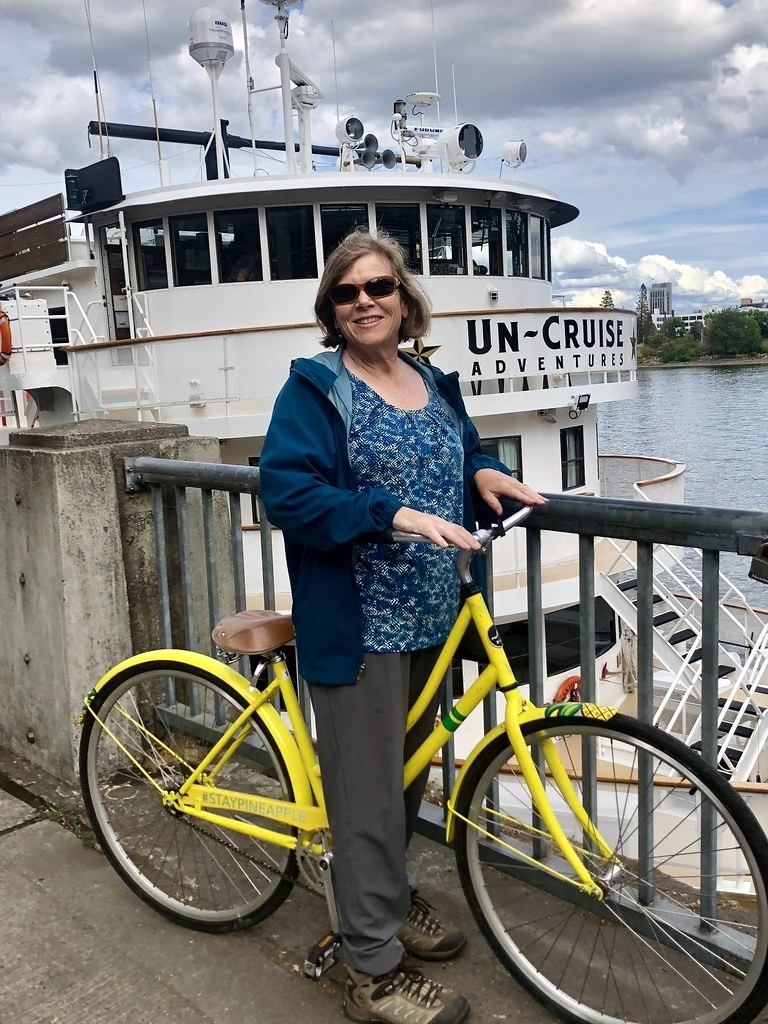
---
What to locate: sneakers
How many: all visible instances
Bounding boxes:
[341,946,471,1024]
[393,883,467,959]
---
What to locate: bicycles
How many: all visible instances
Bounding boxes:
[77,495,768,1024]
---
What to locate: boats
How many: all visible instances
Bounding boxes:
[1,0,768,901]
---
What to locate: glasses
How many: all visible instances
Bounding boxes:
[327,275,401,305]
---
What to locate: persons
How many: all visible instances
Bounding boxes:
[259,225,550,1024]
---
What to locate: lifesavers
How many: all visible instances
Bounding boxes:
[0,308,13,367]
